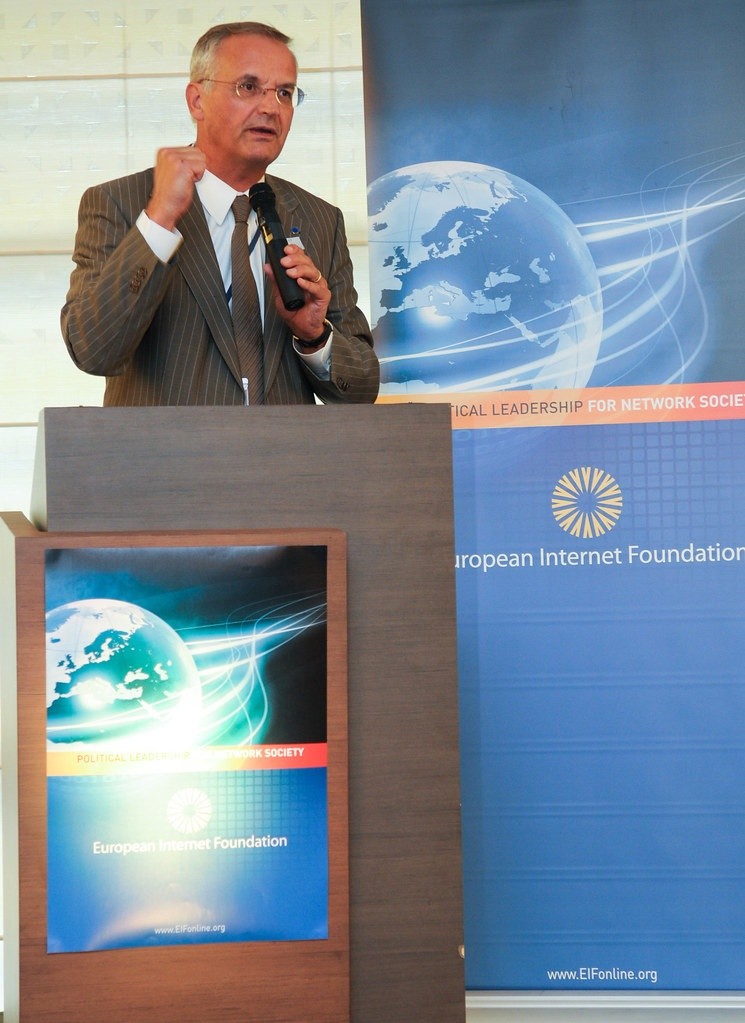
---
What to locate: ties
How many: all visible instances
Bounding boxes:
[231,194,265,405]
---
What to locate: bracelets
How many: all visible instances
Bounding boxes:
[293,320,331,348]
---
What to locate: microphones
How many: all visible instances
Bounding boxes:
[247,180,305,311]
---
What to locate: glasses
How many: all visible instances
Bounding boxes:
[196,78,305,108]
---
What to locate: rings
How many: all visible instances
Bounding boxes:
[315,270,321,282]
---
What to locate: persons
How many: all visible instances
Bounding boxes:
[60,22,380,407]
